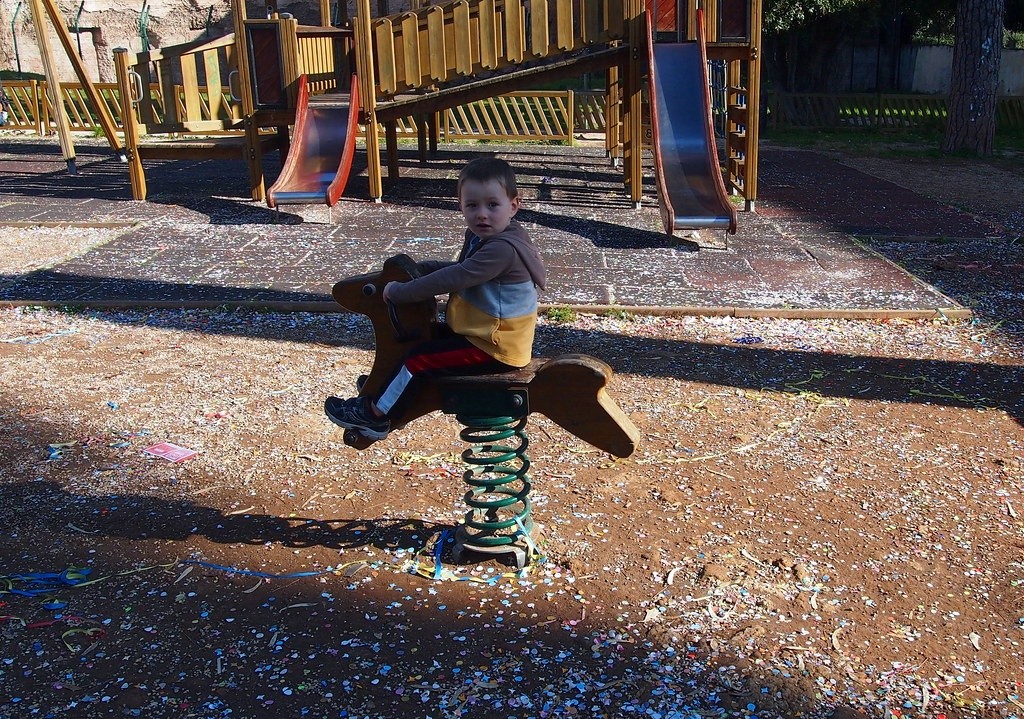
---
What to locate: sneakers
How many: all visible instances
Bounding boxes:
[356,375,369,393]
[324,396,390,440]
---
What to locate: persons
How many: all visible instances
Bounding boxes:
[323,154,550,443]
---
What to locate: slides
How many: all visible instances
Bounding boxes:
[642,40,739,239]
[265,100,358,208]
